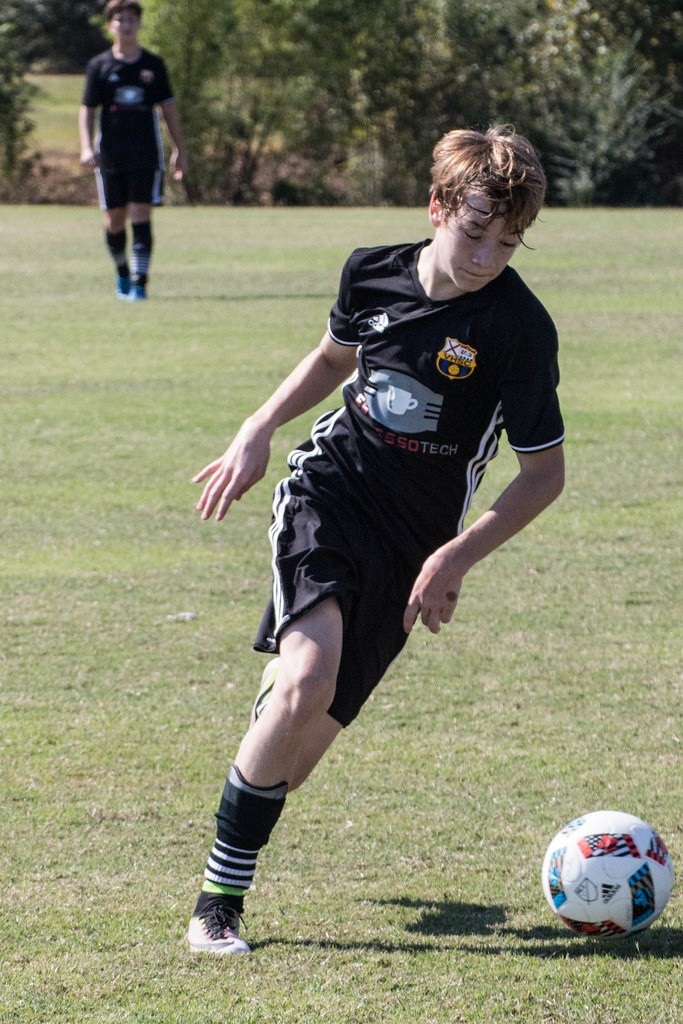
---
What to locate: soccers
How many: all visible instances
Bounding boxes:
[540,810,676,938]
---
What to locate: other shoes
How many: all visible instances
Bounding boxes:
[116,271,131,299]
[131,285,147,303]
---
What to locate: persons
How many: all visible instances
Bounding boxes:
[185,122,567,960]
[75,0,188,301]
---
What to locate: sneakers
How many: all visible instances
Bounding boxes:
[187,893,252,957]
[249,657,280,729]
